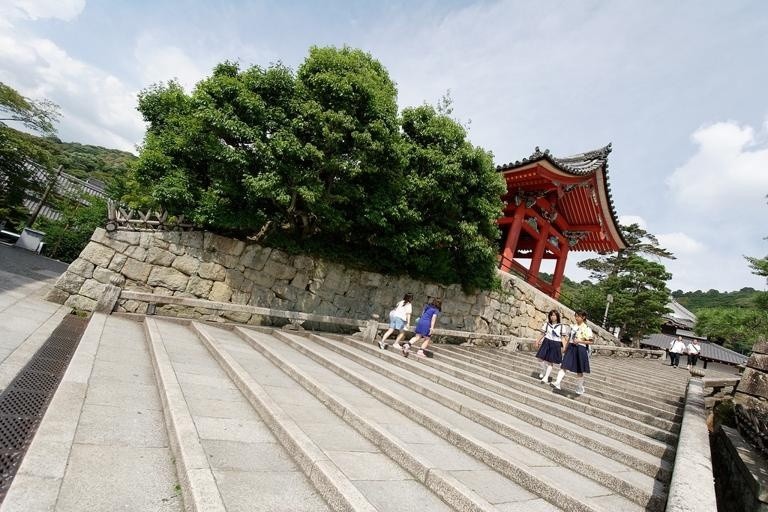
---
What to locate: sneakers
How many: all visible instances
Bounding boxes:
[539,373,561,390]
[378,341,427,357]
[576,385,584,395]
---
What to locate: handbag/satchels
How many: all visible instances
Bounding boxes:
[389,310,395,321]
[414,316,420,327]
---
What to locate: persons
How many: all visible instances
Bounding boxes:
[668,335,687,369]
[550,308,594,394]
[378,292,415,350]
[685,338,701,370]
[534,309,566,383]
[402,298,443,357]
